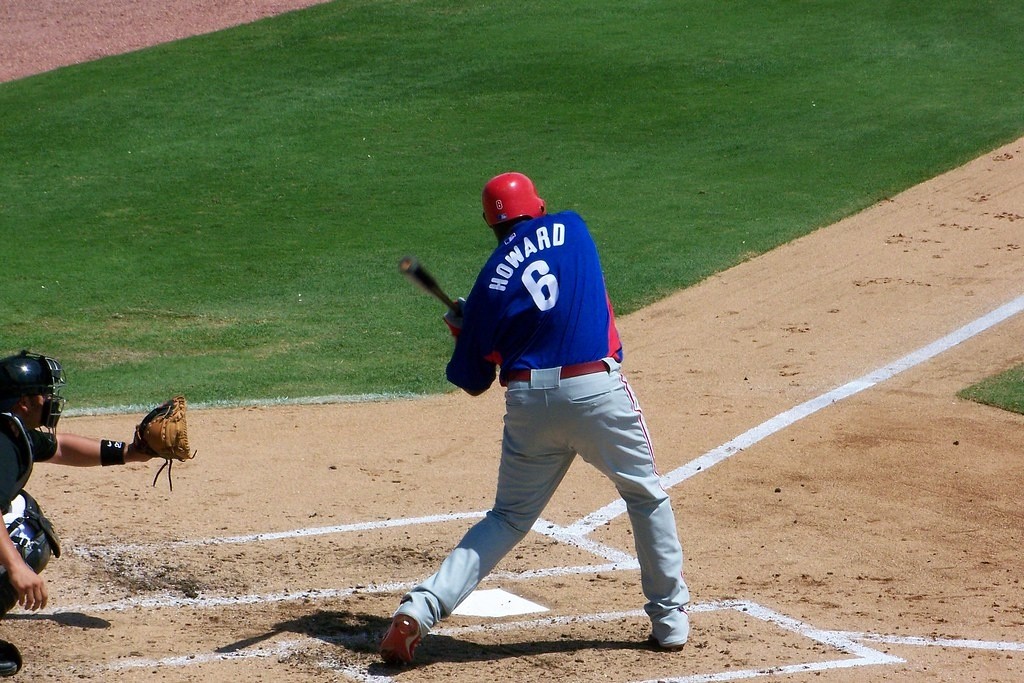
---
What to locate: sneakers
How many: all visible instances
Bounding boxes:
[380,614,423,669]
[652,623,688,648]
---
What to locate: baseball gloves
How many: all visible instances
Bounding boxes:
[132,395,197,493]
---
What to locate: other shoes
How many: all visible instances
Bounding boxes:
[1,663,17,675]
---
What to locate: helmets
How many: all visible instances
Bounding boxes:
[482,172,546,227]
[0,354,53,407]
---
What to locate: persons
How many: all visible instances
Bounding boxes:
[379,172,690,666]
[0,348,197,676]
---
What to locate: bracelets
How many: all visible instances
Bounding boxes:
[100,440,125,467]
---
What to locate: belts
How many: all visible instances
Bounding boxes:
[507,360,609,381]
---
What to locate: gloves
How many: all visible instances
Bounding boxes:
[443,297,466,341]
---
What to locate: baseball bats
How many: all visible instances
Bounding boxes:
[396,254,463,318]
